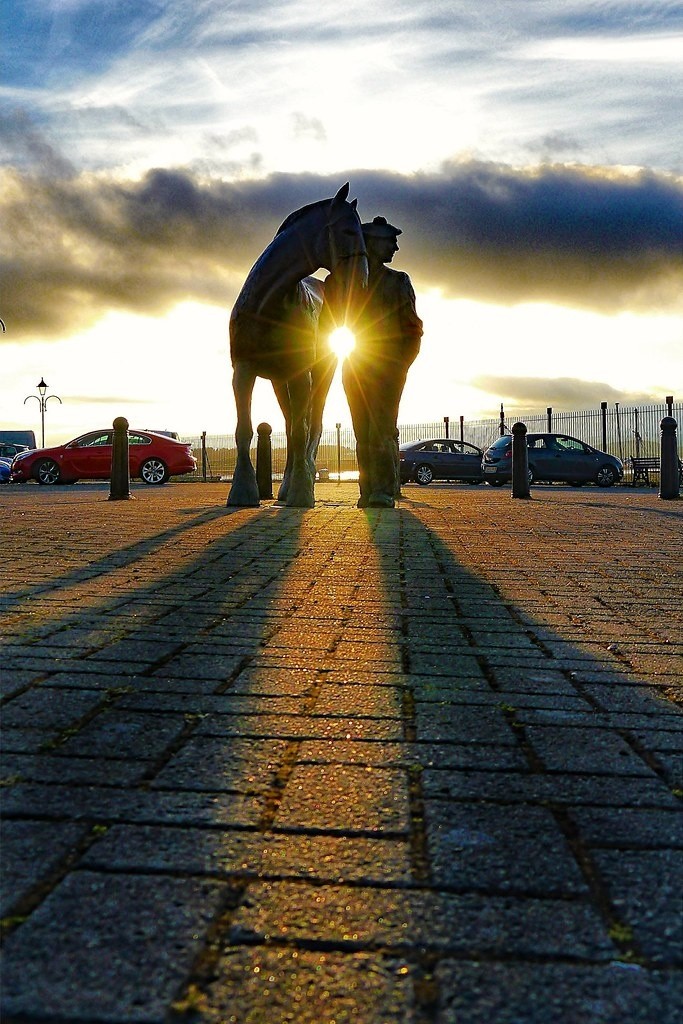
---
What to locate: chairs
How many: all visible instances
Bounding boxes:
[432,446,438,451]
[441,446,447,452]
[529,441,536,448]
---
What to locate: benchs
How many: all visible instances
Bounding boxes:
[630,455,683,487]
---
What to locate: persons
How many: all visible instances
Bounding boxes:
[319,215,424,509]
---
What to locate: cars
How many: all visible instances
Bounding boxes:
[399,438,487,485]
[0,443,30,458]
[481,434,624,487]
[89,429,180,446]
[0,457,13,483]
[623,457,640,468]
[9,429,198,485]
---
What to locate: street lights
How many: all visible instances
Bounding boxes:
[460,416,463,452]
[666,396,673,416]
[601,402,607,452]
[24,377,62,449]
[336,423,341,483]
[444,417,449,439]
[201,431,206,482]
[547,408,552,434]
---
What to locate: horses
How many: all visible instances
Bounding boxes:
[226,181,369,509]
